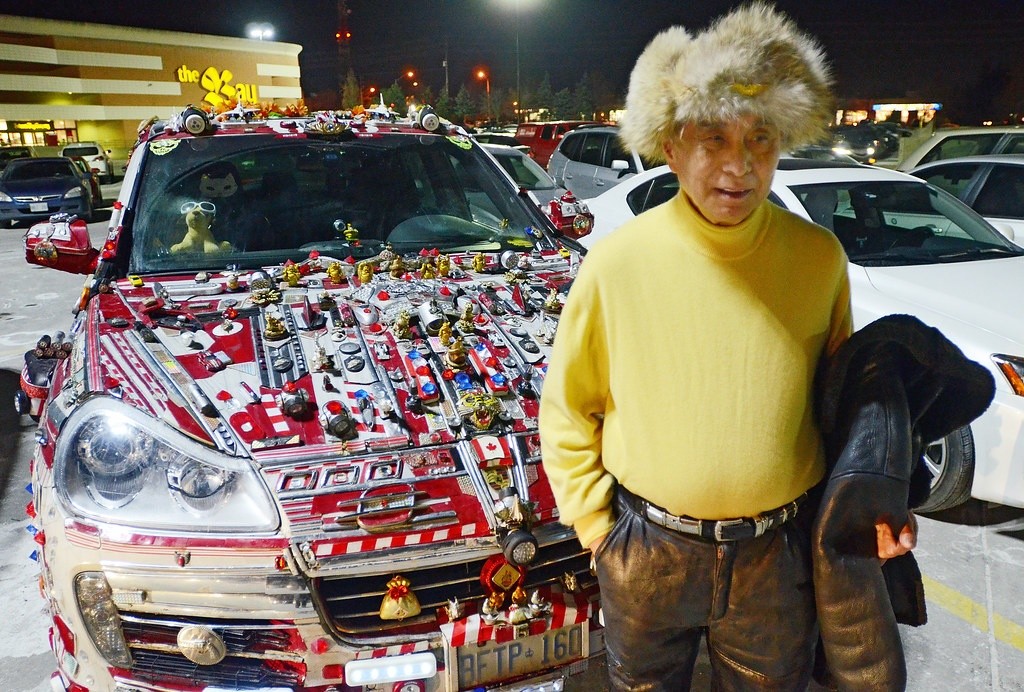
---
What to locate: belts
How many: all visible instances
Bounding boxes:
[616,485,828,542]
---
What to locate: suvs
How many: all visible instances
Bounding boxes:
[509,120,668,202]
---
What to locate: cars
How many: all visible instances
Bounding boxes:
[571,157,1024,516]
[0,141,115,228]
[19,103,595,692]
[833,154,1024,250]
[788,119,911,163]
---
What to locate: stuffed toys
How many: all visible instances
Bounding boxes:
[167,161,245,257]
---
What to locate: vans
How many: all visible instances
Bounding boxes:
[895,125,1024,179]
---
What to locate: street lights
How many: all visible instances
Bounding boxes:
[479,71,491,115]
[394,71,413,85]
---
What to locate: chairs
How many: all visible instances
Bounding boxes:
[328,163,373,202]
[370,170,445,240]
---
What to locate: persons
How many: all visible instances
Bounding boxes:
[538,5,918,692]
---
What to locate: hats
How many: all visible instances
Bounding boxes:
[618,0,843,167]
